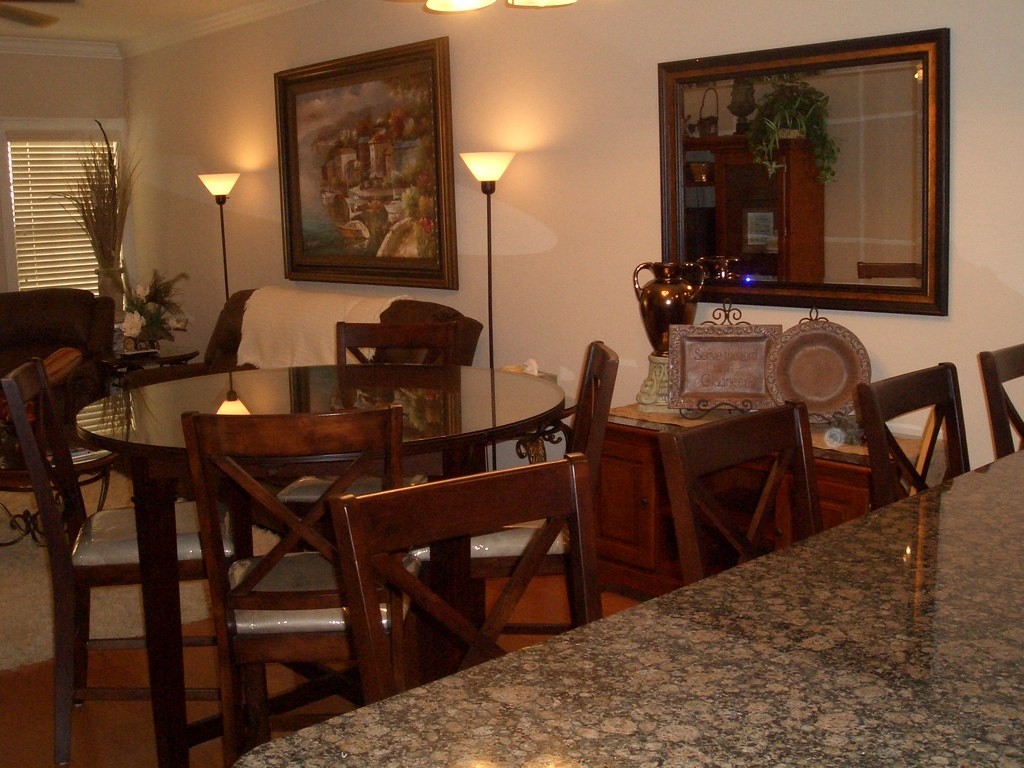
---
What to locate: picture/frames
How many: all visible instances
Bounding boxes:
[669,324,782,409]
[288,365,461,442]
[274,35,460,290]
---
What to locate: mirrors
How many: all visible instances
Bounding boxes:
[657,30,951,320]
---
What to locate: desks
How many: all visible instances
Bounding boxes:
[109,342,197,374]
[233,450,1024,768]
[75,365,567,768]
[0,422,119,547]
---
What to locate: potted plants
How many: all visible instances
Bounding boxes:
[98,269,125,322]
[742,74,840,185]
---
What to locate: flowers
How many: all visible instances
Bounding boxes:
[120,270,189,341]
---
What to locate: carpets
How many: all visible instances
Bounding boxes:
[0,572,642,768]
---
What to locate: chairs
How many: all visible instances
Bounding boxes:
[0,289,619,768]
[658,400,824,586]
[856,364,972,513]
[980,345,1024,459]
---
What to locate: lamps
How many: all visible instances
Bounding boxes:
[196,173,241,300]
[427,0,576,13]
[216,372,251,416]
[457,152,520,369]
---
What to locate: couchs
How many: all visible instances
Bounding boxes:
[0,287,115,423]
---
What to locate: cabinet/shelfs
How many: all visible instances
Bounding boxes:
[680,129,824,283]
[593,402,949,597]
